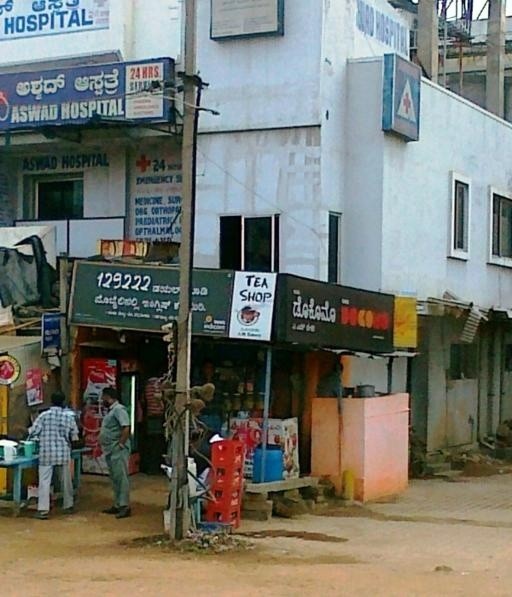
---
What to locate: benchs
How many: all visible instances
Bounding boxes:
[240,477,328,520]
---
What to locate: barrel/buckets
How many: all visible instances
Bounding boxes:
[253,444,282,483]
[357,385,374,398]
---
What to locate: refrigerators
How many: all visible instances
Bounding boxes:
[79,355,146,478]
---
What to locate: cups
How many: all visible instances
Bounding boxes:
[4,445,17,463]
[24,441,34,458]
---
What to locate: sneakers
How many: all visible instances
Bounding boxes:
[30,506,75,520]
[102,503,132,519]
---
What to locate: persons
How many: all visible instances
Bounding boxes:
[27,391,81,519]
[142,361,165,474]
[96,386,134,518]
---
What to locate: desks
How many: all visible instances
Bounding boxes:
[0,446,93,517]
